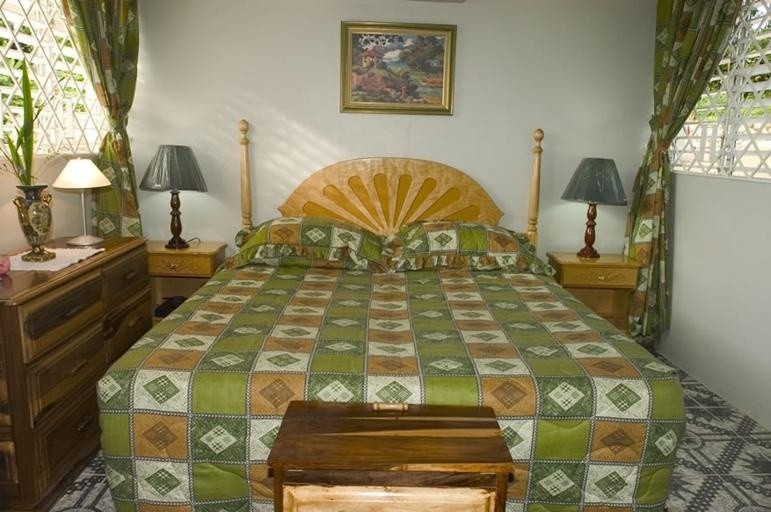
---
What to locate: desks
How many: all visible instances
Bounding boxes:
[264,399,516,512]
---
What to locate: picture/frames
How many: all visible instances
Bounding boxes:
[339,19,458,119]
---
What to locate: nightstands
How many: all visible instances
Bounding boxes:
[146,240,228,327]
[546,251,643,336]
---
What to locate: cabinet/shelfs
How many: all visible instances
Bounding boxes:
[0,233,154,512]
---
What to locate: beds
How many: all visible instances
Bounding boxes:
[97,115,687,511]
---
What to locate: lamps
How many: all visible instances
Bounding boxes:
[52,155,113,246]
[559,157,629,257]
[138,144,208,248]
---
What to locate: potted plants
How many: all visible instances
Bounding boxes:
[0,55,71,262]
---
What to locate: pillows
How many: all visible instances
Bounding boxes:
[387,221,554,274]
[230,216,389,272]
